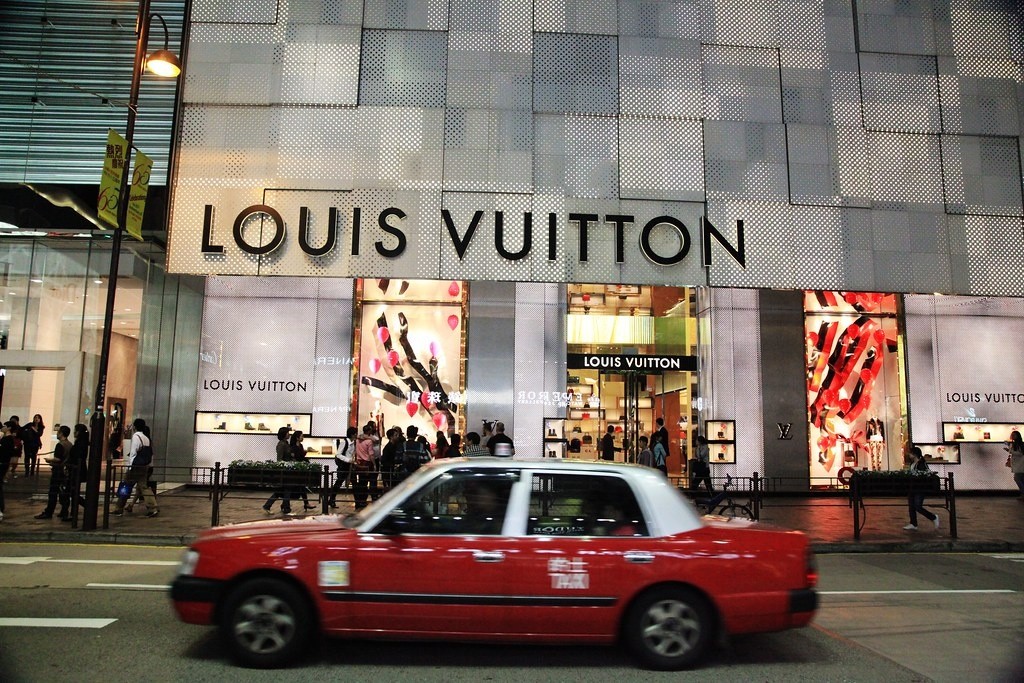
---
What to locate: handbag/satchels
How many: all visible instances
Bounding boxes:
[334,438,348,465]
[568,387,574,395]
[582,412,591,420]
[117,480,130,499]
[127,435,153,466]
[570,439,581,453]
[584,402,590,408]
[614,424,622,432]
[573,387,580,394]
[583,431,592,445]
[572,424,581,432]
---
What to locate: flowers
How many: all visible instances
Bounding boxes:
[853,468,938,481]
[228,459,321,471]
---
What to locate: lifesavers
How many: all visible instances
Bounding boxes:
[837,466,856,486]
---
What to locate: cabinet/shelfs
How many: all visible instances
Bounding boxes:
[567,283,651,316]
[563,369,655,463]
[705,419,736,464]
[543,419,566,458]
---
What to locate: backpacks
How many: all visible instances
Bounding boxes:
[649,443,661,465]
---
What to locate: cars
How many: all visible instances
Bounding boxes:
[166,455,821,672]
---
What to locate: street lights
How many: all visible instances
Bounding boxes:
[81,0,184,533]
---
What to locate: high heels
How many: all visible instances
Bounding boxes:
[304,504,316,512]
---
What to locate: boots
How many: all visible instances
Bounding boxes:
[549,451,557,458]
[548,429,557,436]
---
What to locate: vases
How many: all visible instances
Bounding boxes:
[228,469,321,487]
[849,477,940,497]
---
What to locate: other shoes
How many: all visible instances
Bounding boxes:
[328,499,339,508]
[34,513,52,520]
[125,504,133,512]
[57,511,68,517]
[109,511,123,517]
[145,511,157,517]
[263,506,274,515]
[903,524,917,530]
[932,514,939,529]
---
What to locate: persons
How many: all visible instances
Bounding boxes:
[681,430,717,500]
[262,427,317,516]
[61,423,89,522]
[109,403,124,459]
[482,422,515,457]
[655,418,670,457]
[865,417,886,474]
[328,421,381,512]
[461,432,491,456]
[108,418,160,518]
[34,426,73,519]
[0,414,45,523]
[636,436,653,468]
[443,433,472,458]
[381,425,433,496]
[435,430,449,459]
[649,432,668,477]
[1003,431,1024,501]
[601,424,627,461]
[903,447,939,530]
